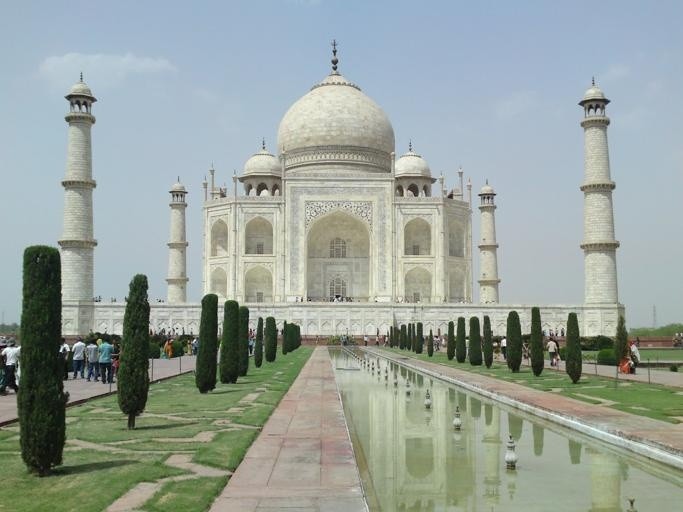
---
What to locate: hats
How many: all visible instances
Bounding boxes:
[549,338,554,340]
[0,339,16,346]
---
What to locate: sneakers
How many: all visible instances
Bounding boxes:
[87,379,116,384]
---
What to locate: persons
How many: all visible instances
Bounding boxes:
[465,337,470,359]
[549,327,564,336]
[374,335,389,346]
[522,340,529,363]
[492,340,499,360]
[433,336,440,350]
[333,294,353,302]
[0,328,256,397]
[625,342,640,375]
[500,336,507,359]
[364,334,368,347]
[316,335,319,344]
[545,338,561,366]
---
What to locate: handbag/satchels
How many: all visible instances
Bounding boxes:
[554,354,561,365]
